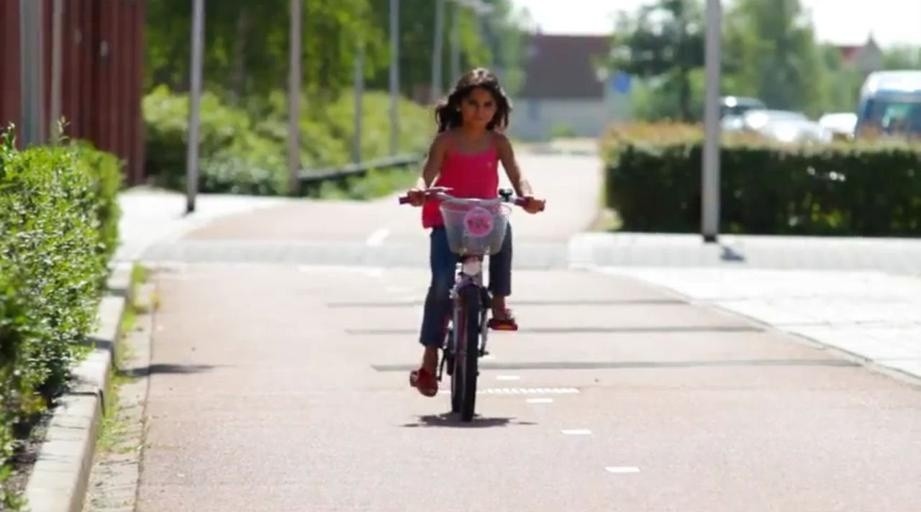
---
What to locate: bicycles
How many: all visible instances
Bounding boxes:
[394,182,544,422]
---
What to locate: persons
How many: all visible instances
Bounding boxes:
[407,67,546,397]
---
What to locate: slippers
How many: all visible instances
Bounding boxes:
[490,314,517,329]
[418,368,437,396]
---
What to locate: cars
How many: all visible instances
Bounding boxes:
[715,71,920,144]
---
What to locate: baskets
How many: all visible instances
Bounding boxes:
[440,199,512,255]
